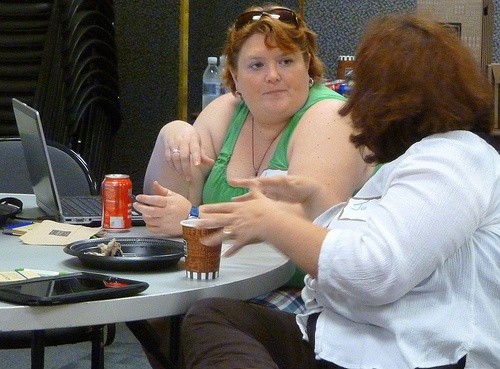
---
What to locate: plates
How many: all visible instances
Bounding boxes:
[63,237,185,270]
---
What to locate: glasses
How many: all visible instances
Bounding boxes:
[235,9,302,32]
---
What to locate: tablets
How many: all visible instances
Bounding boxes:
[0,272,149,306]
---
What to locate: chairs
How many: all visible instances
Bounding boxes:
[0,0,121,345]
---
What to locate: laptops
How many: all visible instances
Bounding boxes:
[13,99,147,226]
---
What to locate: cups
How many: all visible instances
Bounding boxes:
[180,219,224,280]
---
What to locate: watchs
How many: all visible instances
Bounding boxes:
[185,204,200,220]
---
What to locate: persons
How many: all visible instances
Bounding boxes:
[179,12,500,369]
[125,4,384,369]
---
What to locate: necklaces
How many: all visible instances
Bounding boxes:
[252,116,287,176]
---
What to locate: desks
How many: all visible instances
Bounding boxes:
[0,193,294,369]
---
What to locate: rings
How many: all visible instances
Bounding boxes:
[222,226,234,238]
[171,150,181,153]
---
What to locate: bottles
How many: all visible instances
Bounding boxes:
[202,57,221,109]
[218,55,231,95]
